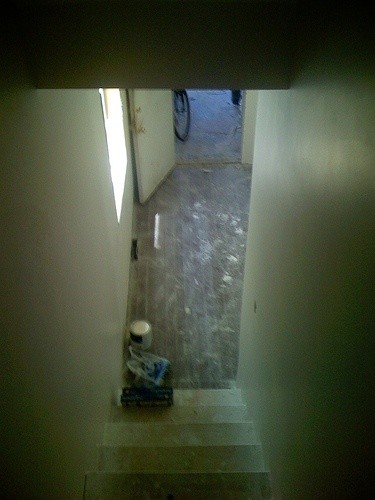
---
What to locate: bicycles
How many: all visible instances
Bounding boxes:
[173,90,192,142]
